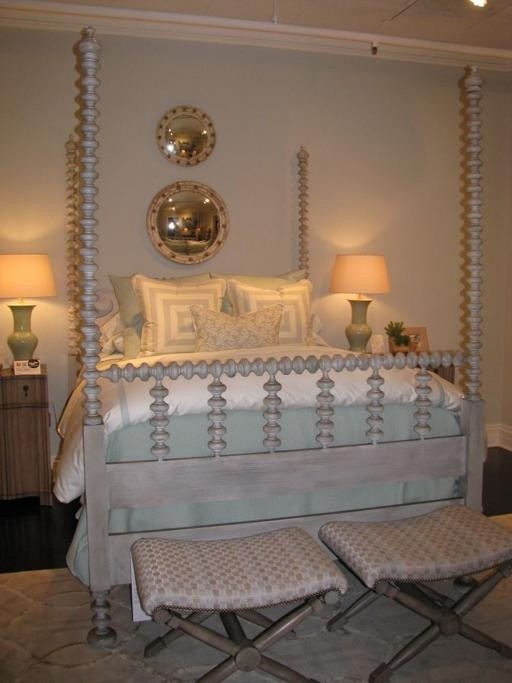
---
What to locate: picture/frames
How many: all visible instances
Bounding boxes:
[389,325,431,355]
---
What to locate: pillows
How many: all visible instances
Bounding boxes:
[108,263,316,358]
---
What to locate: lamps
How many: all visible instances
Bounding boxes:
[328,253,391,353]
[0,253,58,369]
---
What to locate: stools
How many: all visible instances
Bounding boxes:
[132,523,348,683]
[317,505,511,682]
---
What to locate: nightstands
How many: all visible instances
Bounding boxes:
[403,350,456,383]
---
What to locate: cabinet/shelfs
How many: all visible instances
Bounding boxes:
[0,365,53,508]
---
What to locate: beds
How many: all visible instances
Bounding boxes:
[62,29,488,648]
